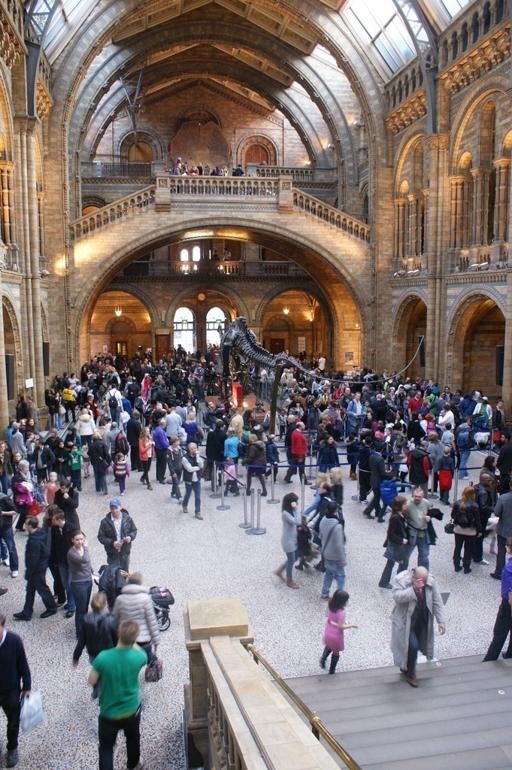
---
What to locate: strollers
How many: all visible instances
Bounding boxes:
[89,563,177,637]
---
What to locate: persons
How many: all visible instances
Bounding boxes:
[169,157,245,195]
[0,613,32,768]
[88,620,149,769]
[0,345,512,688]
[222,248,232,274]
[210,250,220,261]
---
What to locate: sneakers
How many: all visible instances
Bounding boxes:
[378,583,394,589]
[69,477,205,521]
[130,757,145,770]
[406,675,419,687]
[475,558,491,565]
[398,471,471,505]
[489,572,503,580]
[0,557,74,621]
[399,665,408,673]
[320,596,333,602]
[295,562,305,570]
[5,747,20,768]
[212,470,385,523]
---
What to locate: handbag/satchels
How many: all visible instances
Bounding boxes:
[144,659,164,683]
[383,539,412,563]
[315,559,326,573]
[445,519,455,534]
[19,691,46,735]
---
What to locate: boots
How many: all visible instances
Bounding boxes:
[462,558,472,573]
[329,654,340,675]
[319,647,332,668]
[454,558,462,572]
[285,576,300,589]
[275,565,286,583]
